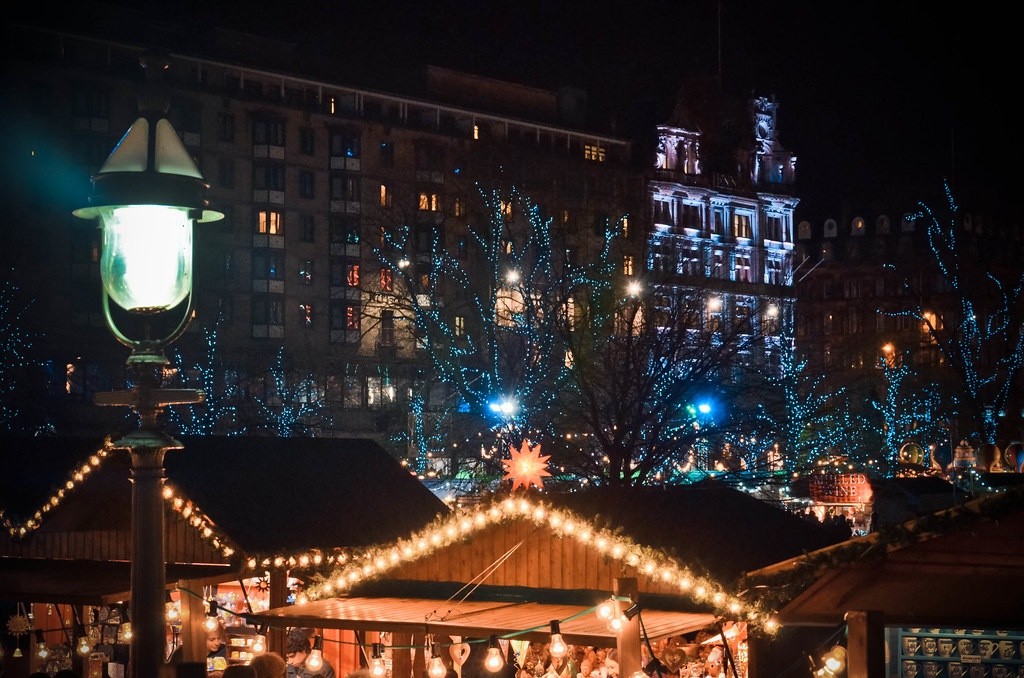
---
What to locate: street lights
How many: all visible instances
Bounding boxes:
[70,44,226,678]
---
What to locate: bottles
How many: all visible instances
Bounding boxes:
[90,652,105,678]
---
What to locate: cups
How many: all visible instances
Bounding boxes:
[999,641,1024,660]
[938,639,958,657]
[232,651,253,660]
[921,661,943,678]
[903,637,922,656]
[922,638,938,656]
[958,638,977,657]
[902,660,921,678]
[207,657,227,671]
[969,663,990,678]
[247,638,253,646]
[231,639,245,646]
[991,664,1024,678]
[908,628,1009,636]
[977,640,999,659]
[947,662,969,678]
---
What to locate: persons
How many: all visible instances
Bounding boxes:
[286,632,335,678]
[251,653,286,678]
[222,665,255,678]
[796,508,852,538]
[171,617,226,678]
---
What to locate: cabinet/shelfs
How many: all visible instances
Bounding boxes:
[226,632,267,668]
[884,627,1024,678]
